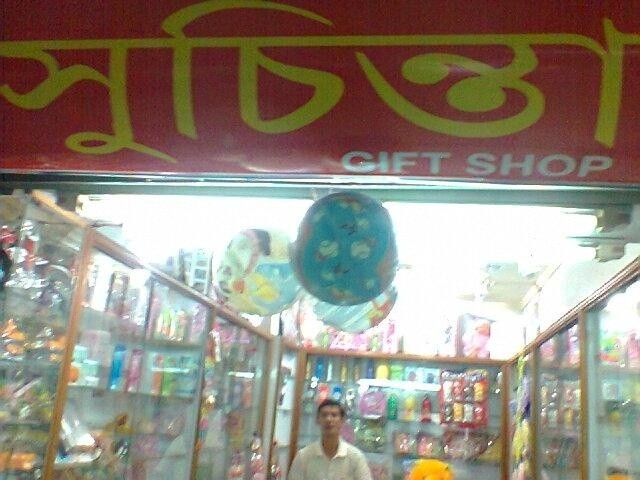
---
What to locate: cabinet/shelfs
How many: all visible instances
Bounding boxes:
[0,188,274,480]
[287,347,505,480]
[505,255,640,480]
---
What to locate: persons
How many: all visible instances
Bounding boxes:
[286,398,372,480]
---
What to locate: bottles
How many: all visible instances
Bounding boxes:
[353,359,360,385]
[318,384,328,401]
[404,394,413,422]
[422,394,431,422]
[366,360,374,380]
[151,355,164,395]
[340,356,347,383]
[331,386,341,401]
[387,393,397,420]
[316,357,323,382]
[325,358,333,382]
[125,348,143,393]
[108,343,125,391]
